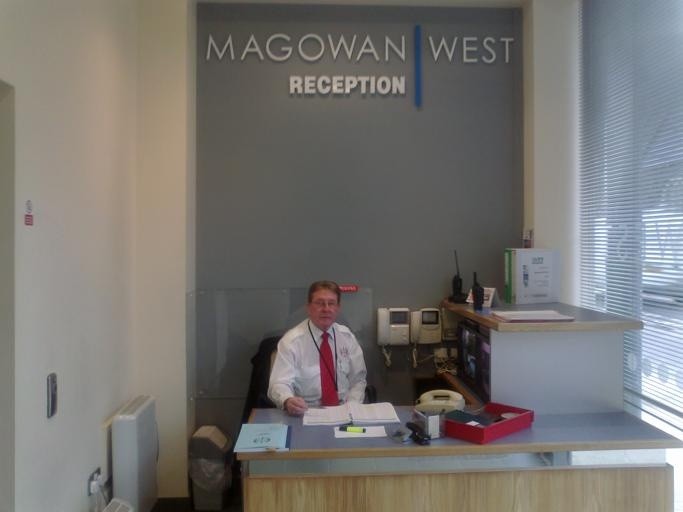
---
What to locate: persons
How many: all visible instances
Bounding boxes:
[265,280,367,417]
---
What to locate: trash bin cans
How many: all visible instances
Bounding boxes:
[188,424,235,512]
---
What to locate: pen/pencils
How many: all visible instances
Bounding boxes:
[339,426,366,433]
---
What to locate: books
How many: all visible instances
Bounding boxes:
[487,309,576,324]
[501,247,562,303]
[302,396,400,426]
[233,422,292,453]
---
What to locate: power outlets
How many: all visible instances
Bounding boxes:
[87,467,102,496]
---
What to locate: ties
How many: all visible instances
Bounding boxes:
[317,331,340,407]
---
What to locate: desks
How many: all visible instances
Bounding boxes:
[235,407,683,511]
[443,298,643,413]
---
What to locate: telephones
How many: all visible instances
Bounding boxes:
[449,275,468,303]
[415,390,465,415]
[376,307,410,346]
[410,308,442,345]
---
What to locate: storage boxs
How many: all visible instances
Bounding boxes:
[443,404,534,443]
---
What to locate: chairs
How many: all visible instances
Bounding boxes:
[241,335,377,422]
